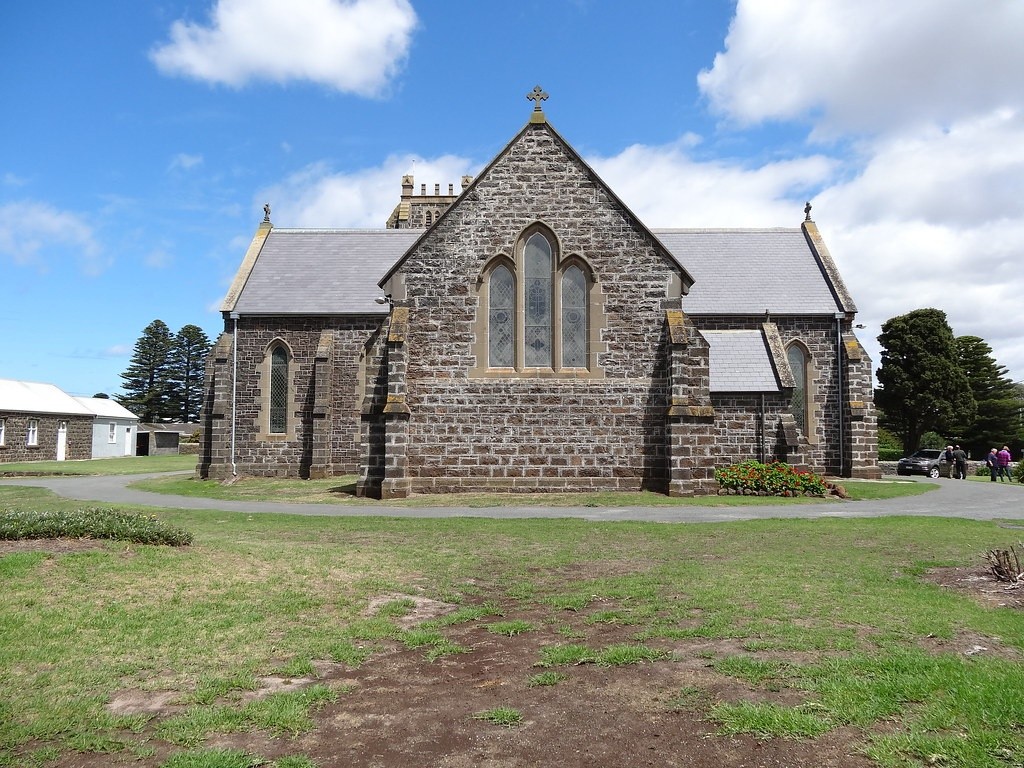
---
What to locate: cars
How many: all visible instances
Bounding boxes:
[896,448,970,479]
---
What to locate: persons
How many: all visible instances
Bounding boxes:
[987,446,1012,482]
[946,446,967,479]
